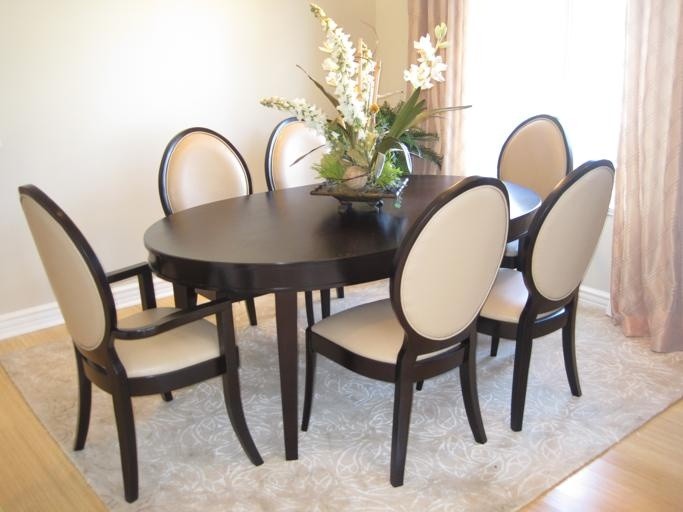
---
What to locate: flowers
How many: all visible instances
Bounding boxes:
[262,3,472,189]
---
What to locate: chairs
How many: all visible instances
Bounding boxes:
[490,113,574,358]
[414,158,617,430]
[267,117,346,327]
[16,183,264,503]
[159,127,259,330]
[300,173,513,488]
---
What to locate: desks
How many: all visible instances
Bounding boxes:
[143,175,543,461]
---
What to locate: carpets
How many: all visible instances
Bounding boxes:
[1,291,681,512]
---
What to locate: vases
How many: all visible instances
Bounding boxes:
[309,174,409,226]
